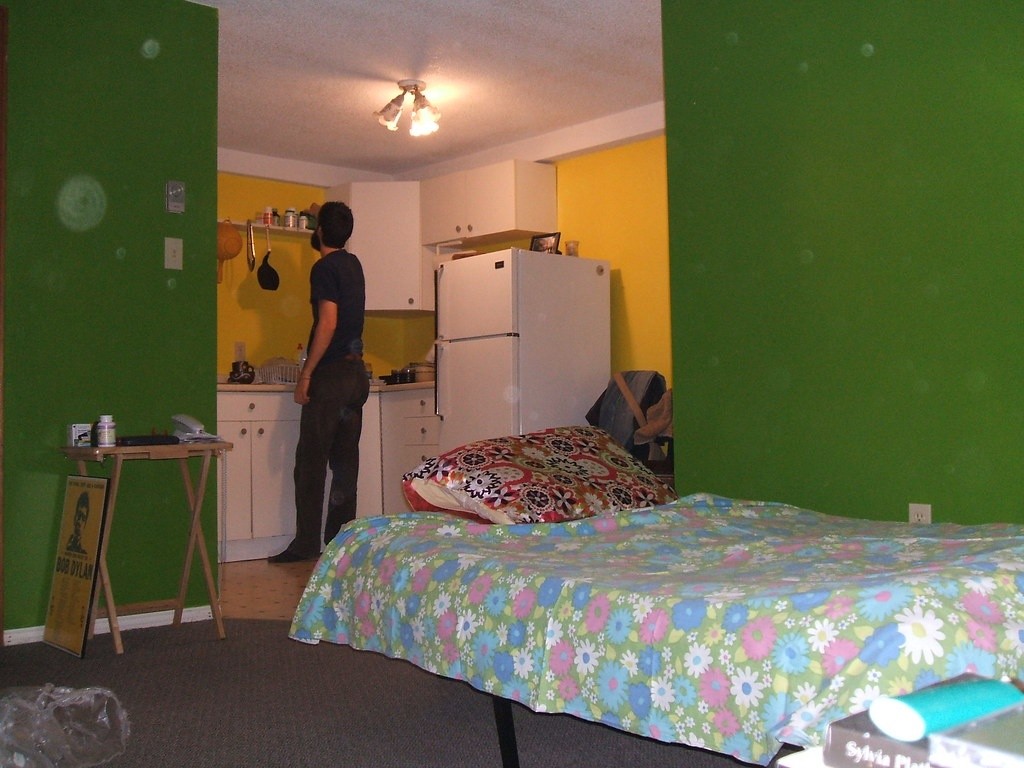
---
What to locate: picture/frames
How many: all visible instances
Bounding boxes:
[529,231,562,254]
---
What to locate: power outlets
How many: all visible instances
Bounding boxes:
[164,237,184,271]
[233,341,246,361]
[908,503,931,524]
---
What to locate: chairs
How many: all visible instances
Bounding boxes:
[585,370,675,489]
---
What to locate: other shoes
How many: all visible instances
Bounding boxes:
[268,551,309,562]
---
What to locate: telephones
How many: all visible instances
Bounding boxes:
[171,413,220,443]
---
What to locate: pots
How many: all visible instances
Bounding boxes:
[215,217,242,284]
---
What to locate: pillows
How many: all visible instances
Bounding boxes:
[403,429,681,525]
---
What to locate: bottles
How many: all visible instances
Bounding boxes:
[263,207,273,227]
[299,211,308,229]
[390,369,402,384]
[284,209,296,228]
[401,368,415,383]
[273,208,281,226]
[97,414,117,447]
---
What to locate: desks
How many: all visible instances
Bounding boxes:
[54,440,235,654]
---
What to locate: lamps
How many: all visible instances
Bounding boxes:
[373,79,442,138]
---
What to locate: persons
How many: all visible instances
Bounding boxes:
[268,200,369,563]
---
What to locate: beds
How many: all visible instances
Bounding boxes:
[284,426,1024,768]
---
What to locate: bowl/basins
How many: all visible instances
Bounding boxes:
[409,362,435,382]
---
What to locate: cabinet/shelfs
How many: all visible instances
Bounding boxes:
[216,388,442,564]
[324,159,556,311]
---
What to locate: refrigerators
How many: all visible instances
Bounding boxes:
[433,247,610,458]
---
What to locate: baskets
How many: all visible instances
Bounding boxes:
[257,365,301,385]
